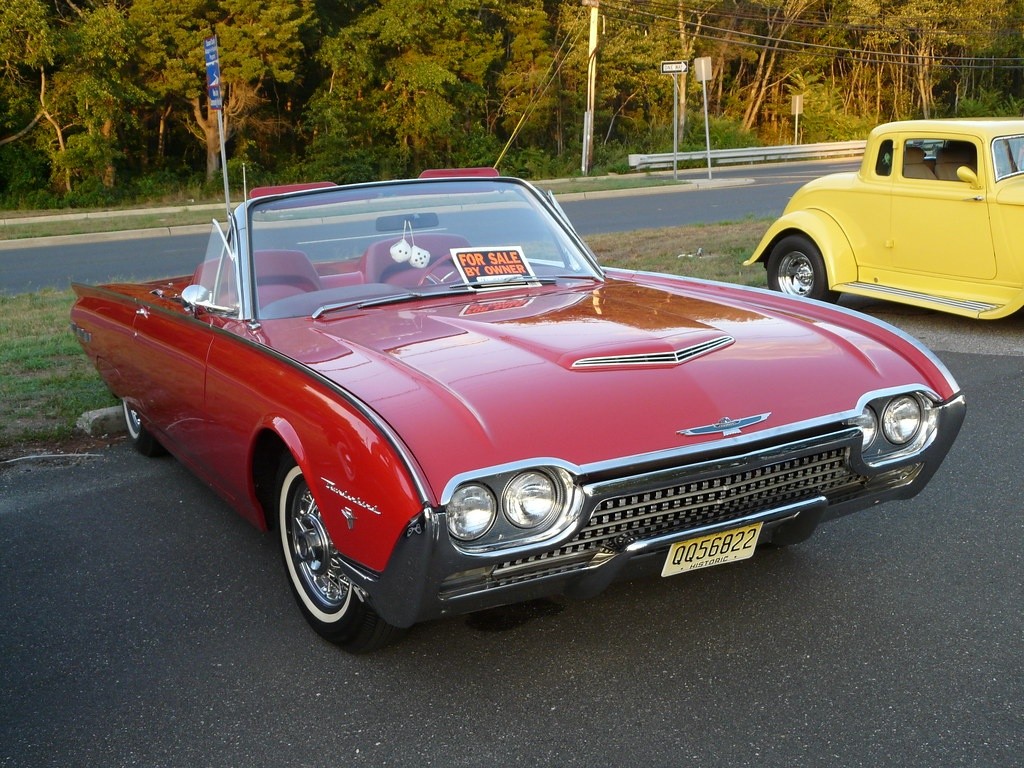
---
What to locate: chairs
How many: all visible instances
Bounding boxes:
[934,148,976,180]
[361,234,470,286]
[888,147,938,180]
[188,249,323,310]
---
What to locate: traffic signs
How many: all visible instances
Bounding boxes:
[662,60,689,73]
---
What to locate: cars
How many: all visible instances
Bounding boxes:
[741,118,1023,329]
[69,166,971,655]
